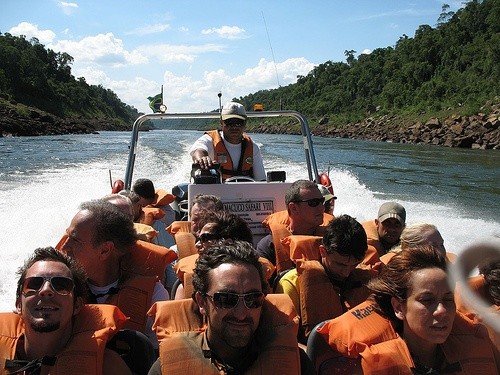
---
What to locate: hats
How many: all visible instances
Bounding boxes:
[221,101,247,121]
[317,184,337,205]
[377,201,406,225]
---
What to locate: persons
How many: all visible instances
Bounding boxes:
[190,101,266,181]
[0,247,131,375]
[149,239,316,375]
[322,245,500,375]
[56,177,500,342]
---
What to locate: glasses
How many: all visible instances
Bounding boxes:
[195,232,216,243]
[205,290,265,311]
[19,276,75,296]
[295,196,325,207]
[223,119,245,128]
